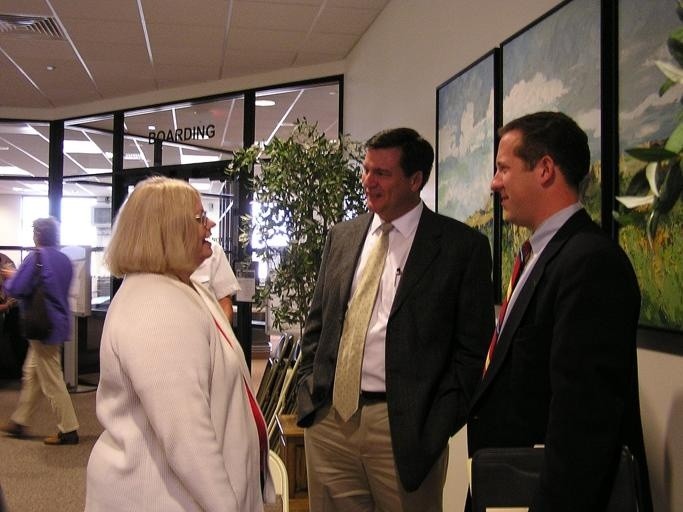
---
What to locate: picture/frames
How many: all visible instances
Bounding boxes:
[436,0,683,353]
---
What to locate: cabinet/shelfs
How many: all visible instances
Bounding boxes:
[273,413,309,509]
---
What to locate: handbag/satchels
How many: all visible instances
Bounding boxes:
[21,249,53,340]
[463,433,644,511]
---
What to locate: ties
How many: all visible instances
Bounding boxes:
[481,239,532,382]
[330,223,396,424]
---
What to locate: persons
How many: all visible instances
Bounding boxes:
[0,215,85,446]
[460,108,658,512]
[182,232,241,329]
[0,296,18,389]
[83,170,285,511]
[295,124,498,510]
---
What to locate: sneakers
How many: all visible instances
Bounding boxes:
[42,429,82,446]
[0,422,26,437]
